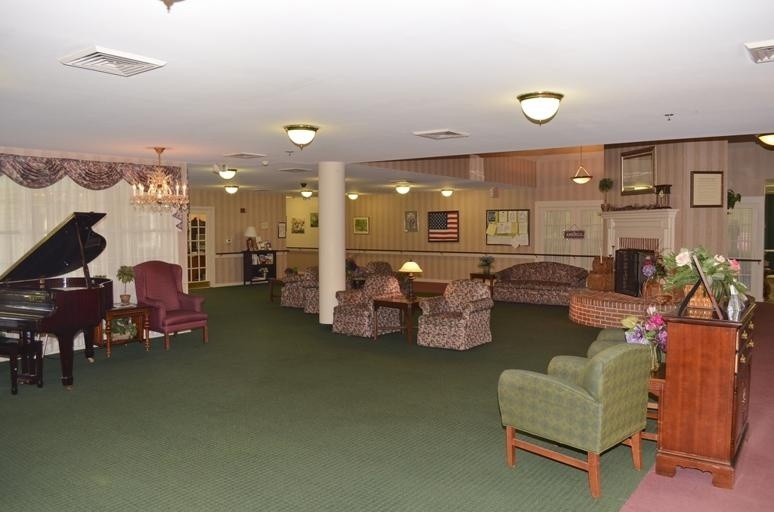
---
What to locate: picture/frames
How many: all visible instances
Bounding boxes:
[681,280,718,324]
[402,208,418,233]
[426,208,462,246]
[350,215,371,236]
[689,168,724,209]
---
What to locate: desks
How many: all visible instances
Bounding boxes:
[263,275,287,306]
[639,361,667,443]
[98,301,153,359]
[366,293,425,346]
[470,271,494,289]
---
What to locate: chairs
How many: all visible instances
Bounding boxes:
[277,259,392,316]
[415,278,496,350]
[129,257,215,351]
[495,340,652,500]
[578,320,658,445]
[331,271,403,340]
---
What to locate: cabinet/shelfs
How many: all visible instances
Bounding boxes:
[240,248,280,286]
[653,293,758,490]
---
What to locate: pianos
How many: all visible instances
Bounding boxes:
[1,211,112,386]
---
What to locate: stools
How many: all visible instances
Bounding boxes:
[0,336,50,396]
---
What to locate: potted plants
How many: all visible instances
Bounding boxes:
[115,264,138,305]
[478,254,496,275]
[595,177,614,213]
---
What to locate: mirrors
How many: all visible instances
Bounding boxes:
[618,143,657,196]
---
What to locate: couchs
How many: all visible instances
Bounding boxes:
[488,261,590,307]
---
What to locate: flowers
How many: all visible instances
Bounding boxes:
[660,246,742,294]
[641,256,667,283]
[618,301,669,373]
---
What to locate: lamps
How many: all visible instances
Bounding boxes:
[439,188,454,199]
[343,191,362,202]
[395,261,421,303]
[280,119,320,148]
[223,184,238,197]
[298,188,315,202]
[515,88,564,127]
[127,145,189,213]
[390,181,412,196]
[566,144,593,185]
[217,164,236,180]
[754,133,773,146]
[242,223,258,251]
